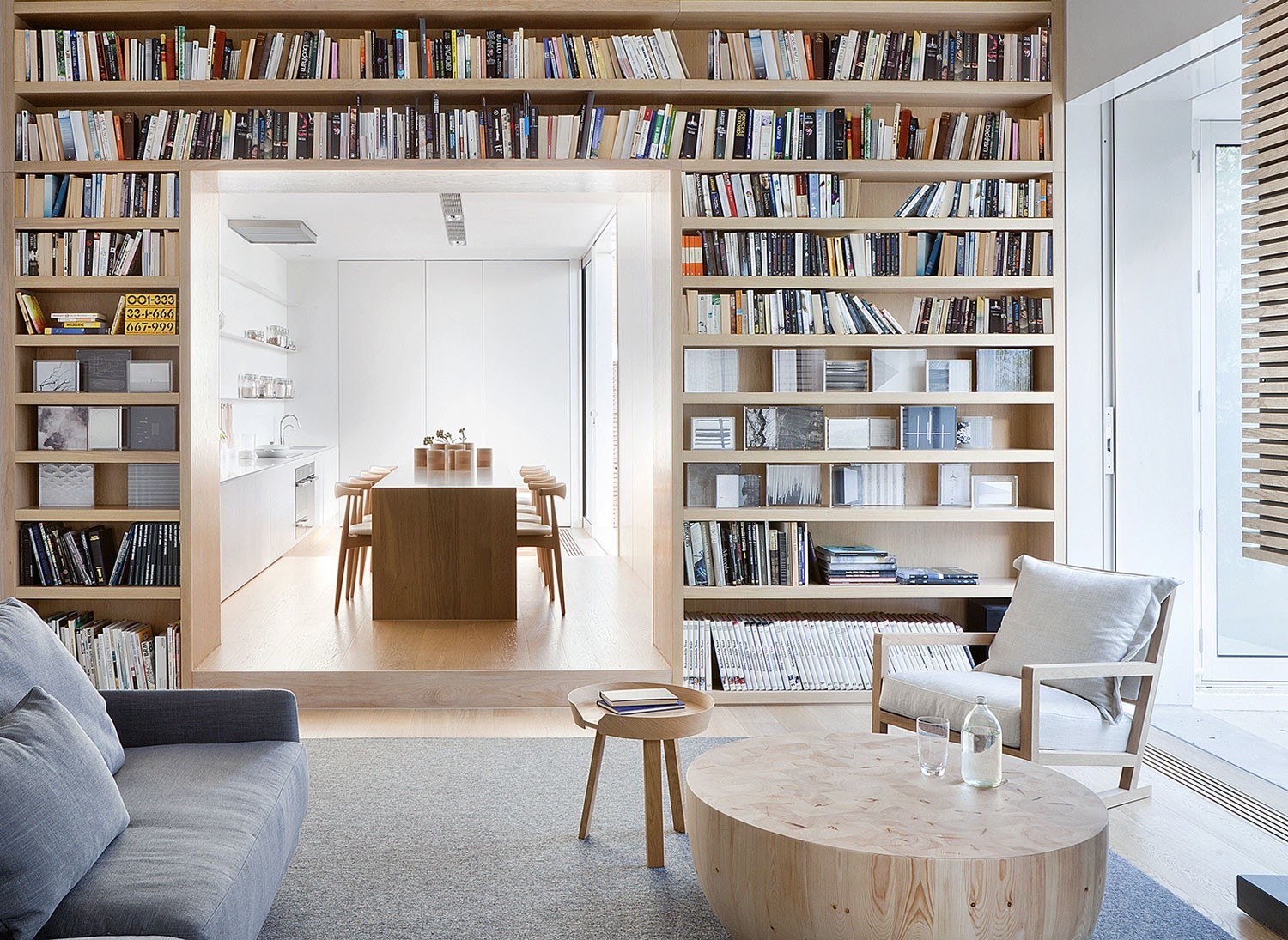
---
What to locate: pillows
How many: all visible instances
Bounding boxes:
[0,686,130,940]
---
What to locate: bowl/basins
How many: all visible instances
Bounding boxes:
[254,442,291,458]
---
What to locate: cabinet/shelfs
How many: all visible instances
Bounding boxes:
[0,0,1066,706]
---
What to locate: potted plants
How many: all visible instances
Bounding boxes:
[436,430,466,470]
[423,436,444,449]
[454,427,471,470]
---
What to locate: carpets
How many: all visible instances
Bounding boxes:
[254,738,1236,940]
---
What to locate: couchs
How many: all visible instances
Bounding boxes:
[0,596,310,940]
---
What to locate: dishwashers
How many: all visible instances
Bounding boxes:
[295,462,319,538]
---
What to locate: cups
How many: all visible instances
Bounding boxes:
[477,448,492,467]
[916,717,950,777]
[427,443,475,472]
[414,448,427,467]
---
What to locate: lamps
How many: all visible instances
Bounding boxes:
[228,219,318,244]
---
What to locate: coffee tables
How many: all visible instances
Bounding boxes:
[684,734,1111,940]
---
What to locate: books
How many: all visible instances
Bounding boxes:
[596,688,686,716]
[11,27,1052,695]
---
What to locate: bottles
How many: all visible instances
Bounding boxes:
[248,325,296,351]
[960,696,1002,787]
[242,374,295,399]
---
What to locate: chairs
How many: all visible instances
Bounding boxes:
[369,468,391,571]
[349,475,377,597]
[370,466,399,571]
[359,471,388,585]
[518,465,547,567]
[518,472,551,571]
[517,476,556,585]
[871,560,1194,809]
[518,469,550,572]
[517,483,566,616]
[335,481,372,614]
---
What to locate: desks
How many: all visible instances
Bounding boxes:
[567,683,715,868]
[370,464,517,622]
